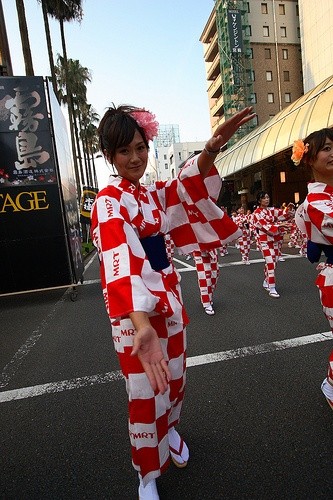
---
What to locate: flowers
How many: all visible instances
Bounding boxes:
[291,138,309,166]
[129,107,159,141]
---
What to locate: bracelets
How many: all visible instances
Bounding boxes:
[204,146,220,155]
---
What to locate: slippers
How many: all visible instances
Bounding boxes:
[299,251,306,256]
[226,252,229,254]
[167,429,189,467]
[322,378,333,408]
[139,482,159,500]
[204,306,215,315]
[242,262,251,265]
[279,257,285,261]
[256,247,260,251]
[221,254,224,256]
[186,255,191,261]
[235,247,240,250]
[295,245,301,249]
[263,283,270,291]
[269,291,280,298]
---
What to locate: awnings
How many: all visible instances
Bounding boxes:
[212,75,333,179]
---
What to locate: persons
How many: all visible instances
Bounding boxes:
[186,191,312,315]
[291,127,333,411]
[88,103,259,500]
